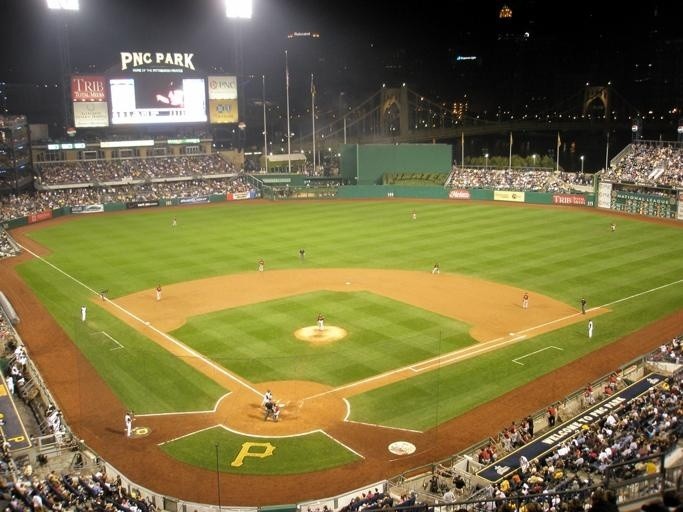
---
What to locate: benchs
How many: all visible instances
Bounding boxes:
[349,493,415,512]
[0,472,156,512]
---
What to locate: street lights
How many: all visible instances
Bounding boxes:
[532,154,536,168]
[484,153,489,170]
[47,1,81,141]
[580,155,585,173]
[223,1,253,155]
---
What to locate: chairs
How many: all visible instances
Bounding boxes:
[490,458,590,512]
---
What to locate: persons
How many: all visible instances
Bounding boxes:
[297,247,305,262]
[0,231,16,259]
[171,216,177,226]
[411,206,416,221]
[522,291,528,310]
[98,286,106,302]
[428,334,683,512]
[578,296,587,315]
[431,262,440,275]
[307,488,416,512]
[261,388,280,423]
[123,411,132,438]
[443,139,683,221]
[79,304,87,324]
[587,319,593,339]
[155,78,183,110]
[608,222,616,232]
[154,283,162,301]
[70,453,164,512]
[255,258,265,272]
[1,128,344,225]
[0,306,70,512]
[316,313,324,331]
